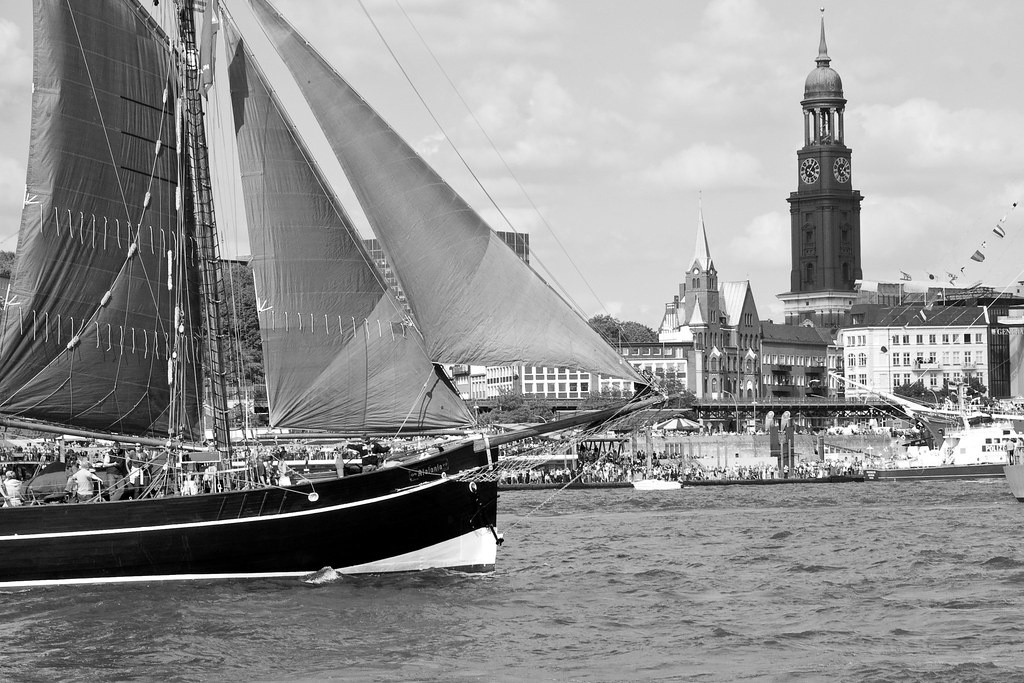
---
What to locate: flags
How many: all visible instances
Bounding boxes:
[971,251,985,262]
[993,225,1005,238]
[915,310,928,325]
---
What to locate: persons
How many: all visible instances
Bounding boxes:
[1,400,1023,509]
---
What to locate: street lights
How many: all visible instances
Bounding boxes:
[723,390,739,434]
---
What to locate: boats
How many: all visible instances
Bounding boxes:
[1004,433,1024,498]
[629,478,682,491]
[836,202,1024,482]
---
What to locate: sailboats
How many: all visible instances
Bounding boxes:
[0,1,684,592]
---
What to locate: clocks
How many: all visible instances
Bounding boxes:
[800,158,820,184]
[833,157,851,183]
